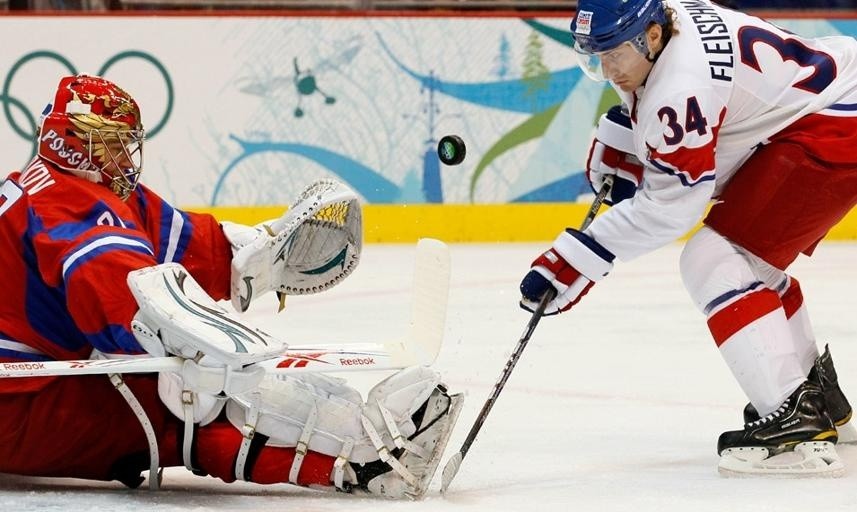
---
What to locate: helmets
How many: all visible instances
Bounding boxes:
[39,73,145,199]
[568,1,671,83]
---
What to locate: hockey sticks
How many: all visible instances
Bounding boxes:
[0,240,453,380]
[440,175,612,491]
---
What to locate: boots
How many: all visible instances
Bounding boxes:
[361,390,449,500]
[716,344,853,457]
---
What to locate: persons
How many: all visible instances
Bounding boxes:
[0,74,450,503]
[518,0,857,454]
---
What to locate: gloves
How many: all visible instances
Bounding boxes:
[517,228,616,315]
[587,103,642,206]
